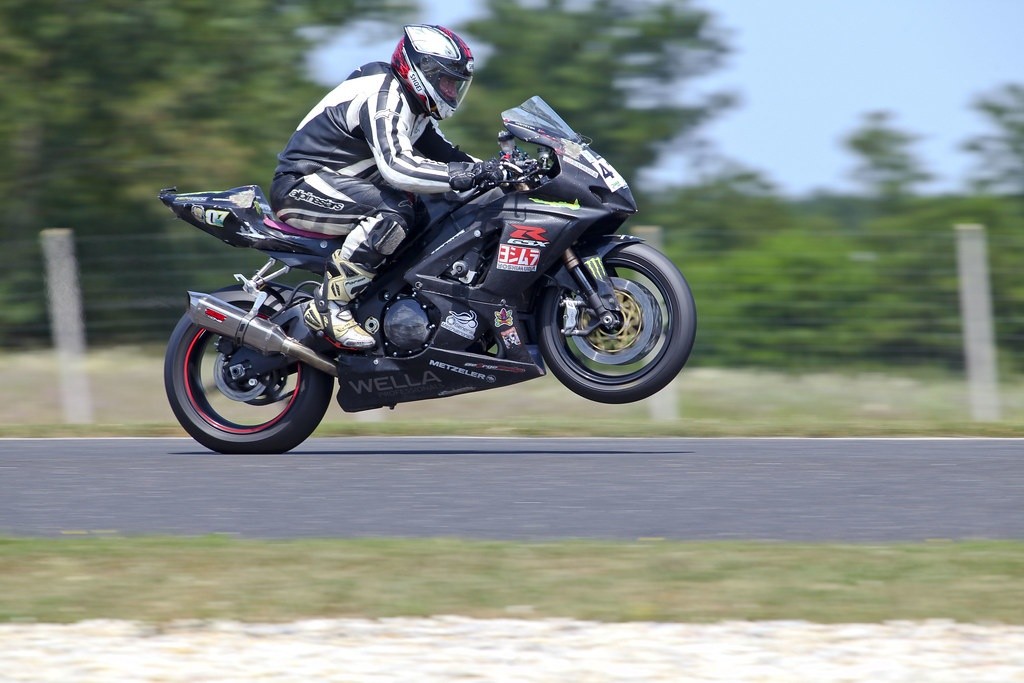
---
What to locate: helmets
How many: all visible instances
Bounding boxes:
[391,22,475,121]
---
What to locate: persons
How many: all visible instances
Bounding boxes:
[270,25,523,350]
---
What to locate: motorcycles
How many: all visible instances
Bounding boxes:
[164,87,698,457]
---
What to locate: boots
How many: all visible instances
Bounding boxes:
[304,249,377,353]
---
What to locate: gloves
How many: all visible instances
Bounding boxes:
[450,160,506,191]
